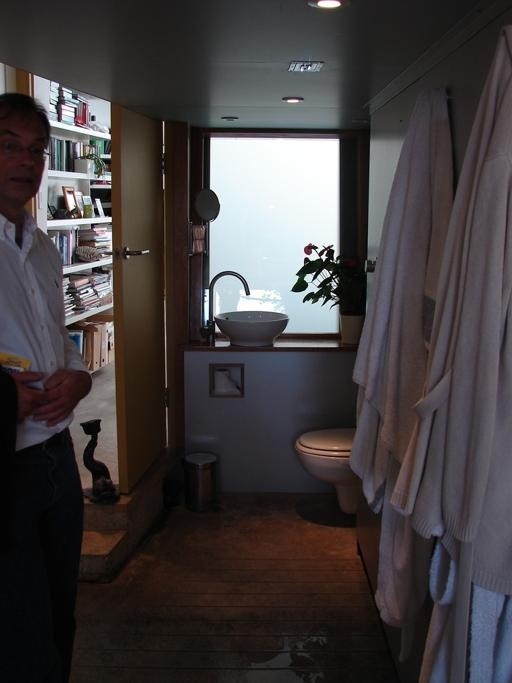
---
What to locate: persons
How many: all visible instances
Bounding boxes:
[1,92,92,681]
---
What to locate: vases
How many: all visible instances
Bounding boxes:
[338,317,362,347]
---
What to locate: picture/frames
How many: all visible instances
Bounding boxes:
[209,362,245,398]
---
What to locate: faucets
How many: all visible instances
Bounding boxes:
[202,271,251,347]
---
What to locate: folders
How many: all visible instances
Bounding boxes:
[75,321,109,370]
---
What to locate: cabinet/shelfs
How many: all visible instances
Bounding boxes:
[44,121,115,376]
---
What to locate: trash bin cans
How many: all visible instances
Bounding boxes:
[186,453,217,513]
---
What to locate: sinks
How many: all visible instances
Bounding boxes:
[214,311,289,347]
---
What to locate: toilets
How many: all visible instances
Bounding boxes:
[294,428,361,516]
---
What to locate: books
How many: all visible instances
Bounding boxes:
[43,77,115,378]
[1,350,31,373]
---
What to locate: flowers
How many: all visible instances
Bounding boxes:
[293,244,385,316]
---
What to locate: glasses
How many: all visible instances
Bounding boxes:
[0,139,52,163]
